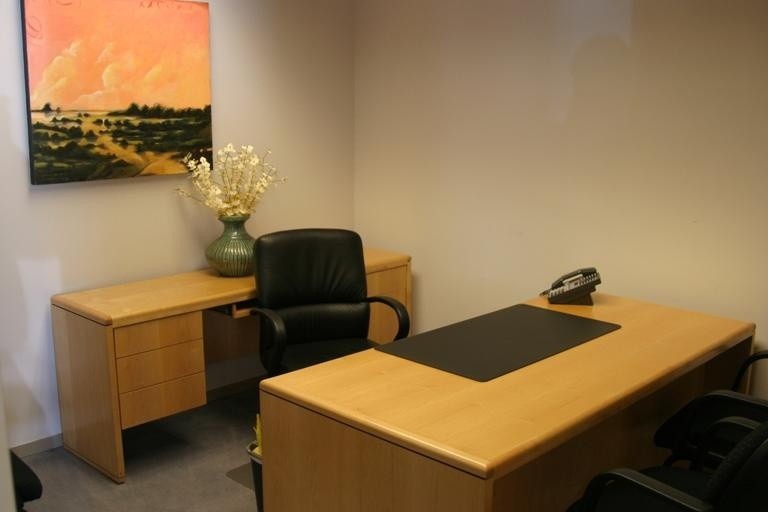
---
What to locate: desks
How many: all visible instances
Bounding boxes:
[258,288,756,512]
[47,243,413,484]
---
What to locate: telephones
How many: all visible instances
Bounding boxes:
[548,267,602,304]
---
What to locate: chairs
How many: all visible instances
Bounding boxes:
[651,348,768,466]
[248,227,411,374]
[563,412,768,511]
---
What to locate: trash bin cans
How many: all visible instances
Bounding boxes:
[246,439,265,512]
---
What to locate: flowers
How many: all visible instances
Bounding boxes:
[173,142,287,220]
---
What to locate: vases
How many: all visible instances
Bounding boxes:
[203,215,259,278]
